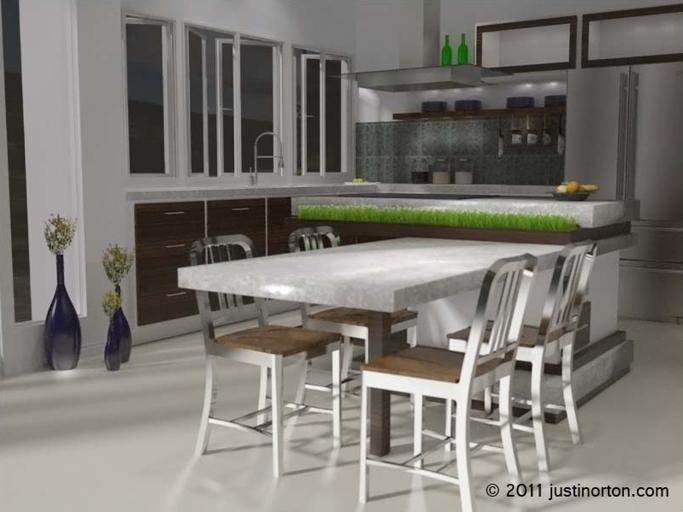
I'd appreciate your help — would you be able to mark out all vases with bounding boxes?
[103,317,120,371]
[105,286,131,363]
[44,255,81,371]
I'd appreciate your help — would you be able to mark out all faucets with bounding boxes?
[248,131,284,185]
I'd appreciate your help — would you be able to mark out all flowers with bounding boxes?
[100,289,122,316]
[39,212,79,254]
[100,239,136,286]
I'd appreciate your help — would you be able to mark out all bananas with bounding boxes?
[580,185,599,191]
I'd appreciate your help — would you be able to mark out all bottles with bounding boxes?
[440,33,470,65]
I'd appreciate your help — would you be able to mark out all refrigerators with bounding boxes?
[566,60,683,324]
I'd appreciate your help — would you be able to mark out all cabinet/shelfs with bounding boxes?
[267,196,291,258]
[134,200,205,325]
[205,197,266,311]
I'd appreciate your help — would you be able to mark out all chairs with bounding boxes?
[443,236,598,469]
[286,224,418,424]
[188,233,345,479]
[357,251,539,512]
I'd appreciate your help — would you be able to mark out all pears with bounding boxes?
[567,181,579,192]
